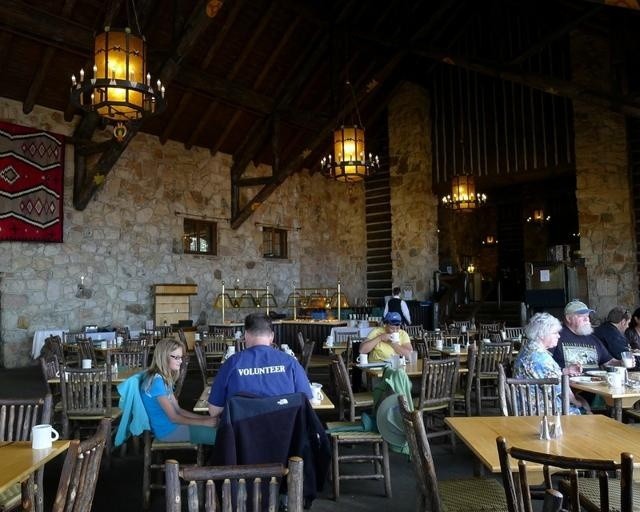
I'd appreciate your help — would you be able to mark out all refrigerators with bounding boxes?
[525,261,588,317]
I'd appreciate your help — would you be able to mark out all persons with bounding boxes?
[623,307,640,349]
[552,300,637,424]
[137,338,223,446]
[383,287,412,325]
[593,304,630,360]
[510,312,593,415]
[356,313,413,416]
[208,313,313,417]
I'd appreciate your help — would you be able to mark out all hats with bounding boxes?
[384,312,401,325]
[566,301,596,314]
[376,393,412,447]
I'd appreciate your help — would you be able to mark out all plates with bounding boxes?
[383,358,409,363]
[585,370,608,376]
[569,376,605,384]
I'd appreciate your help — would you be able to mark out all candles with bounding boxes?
[81,275,84,284]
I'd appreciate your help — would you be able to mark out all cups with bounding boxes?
[408,351,418,364]
[101,341,107,349]
[227,346,236,356]
[454,344,460,352]
[391,356,399,369]
[31,424,59,449]
[461,326,467,332]
[621,351,633,368]
[356,353,368,365]
[117,337,124,343]
[389,332,400,343]
[56,367,70,380]
[607,372,621,388]
[435,339,445,347]
[309,383,323,402]
[286,349,295,356]
[614,366,626,384]
[82,359,92,368]
[281,343,289,351]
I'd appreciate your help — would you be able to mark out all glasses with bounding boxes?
[623,309,630,319]
[170,355,183,360]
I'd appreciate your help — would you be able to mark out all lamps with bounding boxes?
[319,78,381,184]
[528,205,550,227]
[483,234,500,247]
[441,135,487,213]
[69,0,166,141]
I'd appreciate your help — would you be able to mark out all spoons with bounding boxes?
[626,370,633,387]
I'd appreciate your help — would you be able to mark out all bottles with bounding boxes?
[542,415,551,441]
[346,313,369,328]
[553,411,563,436]
